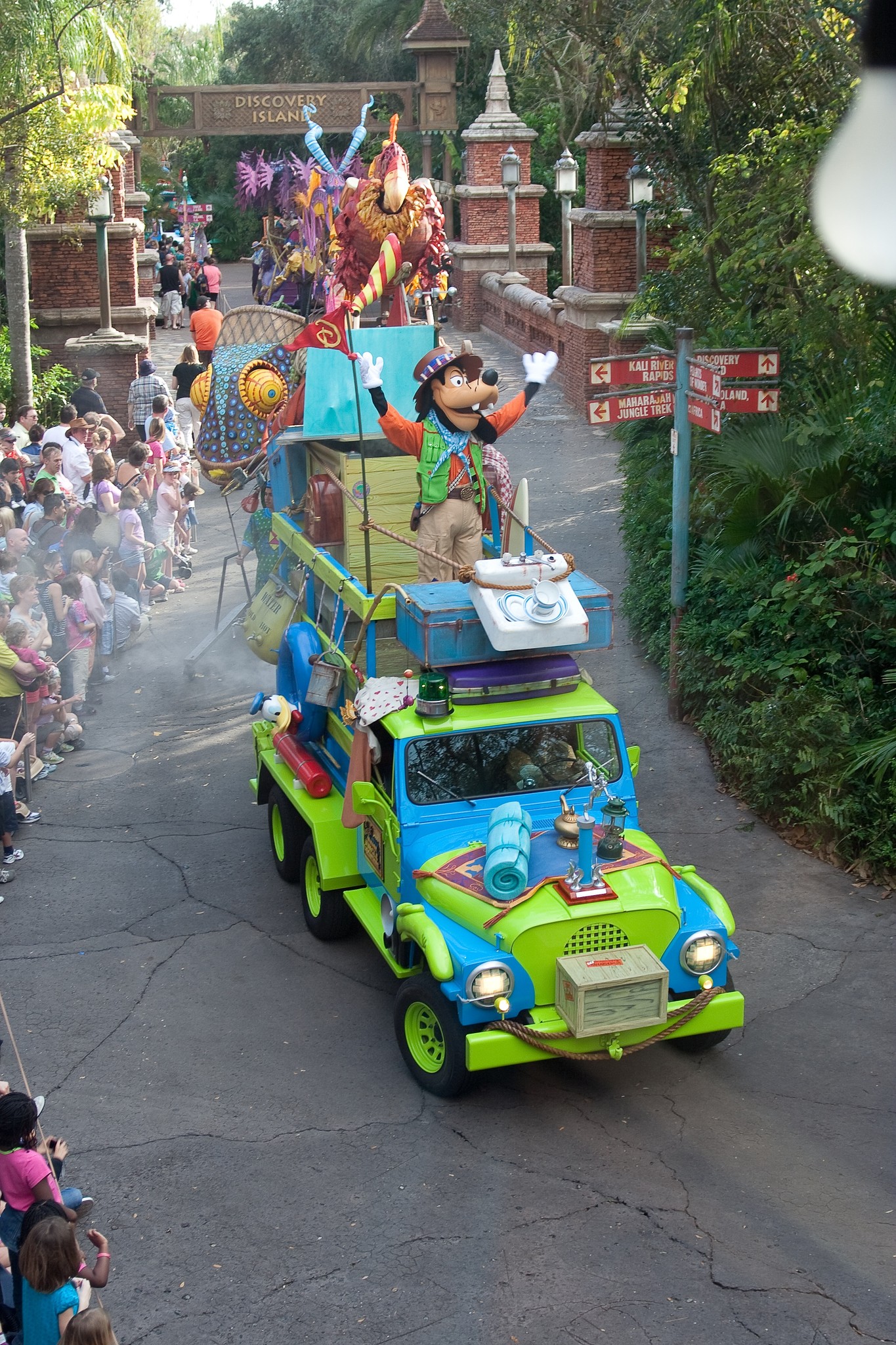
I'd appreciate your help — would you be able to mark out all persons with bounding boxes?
[239,211,303,295]
[0,234,223,904]
[0,1076,119,1345]
[236,478,278,600]
[500,727,585,792]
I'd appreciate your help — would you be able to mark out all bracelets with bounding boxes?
[96,1253,111,1259]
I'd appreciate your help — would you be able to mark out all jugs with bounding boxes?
[554,795,582,850]
[304,650,346,709]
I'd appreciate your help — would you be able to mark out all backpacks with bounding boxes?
[22,520,59,556]
[197,273,208,294]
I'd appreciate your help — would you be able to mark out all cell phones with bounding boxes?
[49,1140,61,1148]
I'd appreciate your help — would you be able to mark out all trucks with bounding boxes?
[249,503,748,1102]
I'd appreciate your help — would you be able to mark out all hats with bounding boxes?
[81,368,100,380]
[184,482,205,495]
[173,455,192,463]
[0,427,20,440]
[162,465,182,472]
[65,418,95,439]
[138,360,157,376]
[251,240,261,249]
[0,1092,45,1145]
[196,295,210,309]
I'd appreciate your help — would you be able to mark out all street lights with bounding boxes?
[625,154,658,322]
[550,145,580,309]
[494,142,529,279]
[90,174,118,336]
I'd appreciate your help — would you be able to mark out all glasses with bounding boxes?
[29,415,39,419]
[9,438,16,443]
[43,490,54,495]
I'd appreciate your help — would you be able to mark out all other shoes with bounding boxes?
[106,671,120,677]
[140,605,150,612]
[86,697,102,704]
[161,591,168,601]
[75,1197,94,1220]
[184,554,192,559]
[173,557,189,562]
[182,547,198,554]
[162,323,185,330]
[148,600,155,605]
[91,691,104,697]
[72,707,97,716]
[90,675,114,686]
[69,738,85,749]
[78,721,86,729]
[43,764,57,772]
[0,868,15,884]
[38,770,47,779]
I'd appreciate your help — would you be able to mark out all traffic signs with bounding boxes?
[694,348,780,377]
[588,360,676,386]
[176,204,213,213]
[177,214,213,224]
[689,365,723,400]
[587,390,674,424]
[685,397,722,435]
[695,389,781,413]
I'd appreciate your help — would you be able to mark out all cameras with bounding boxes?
[31,611,42,620]
[144,463,151,470]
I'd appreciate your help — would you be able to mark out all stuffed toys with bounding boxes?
[357,337,561,582]
[332,112,450,317]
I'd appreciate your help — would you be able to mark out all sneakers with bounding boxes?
[3,848,24,864]
[19,812,40,823]
[56,744,74,754]
[39,751,65,764]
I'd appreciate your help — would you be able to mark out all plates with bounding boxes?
[524,593,568,624]
[497,591,531,621]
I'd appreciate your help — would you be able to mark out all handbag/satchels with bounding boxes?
[92,509,121,548]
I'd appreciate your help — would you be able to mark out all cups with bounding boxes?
[530,578,561,614]
